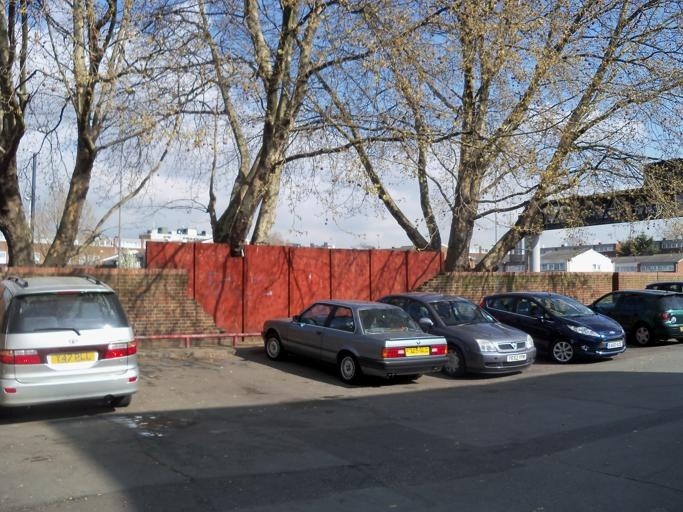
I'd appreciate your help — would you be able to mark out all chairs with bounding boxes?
[13,297,115,333]
[493,298,562,315]
[411,304,458,327]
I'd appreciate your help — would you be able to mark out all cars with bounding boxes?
[0,273,138,406]
